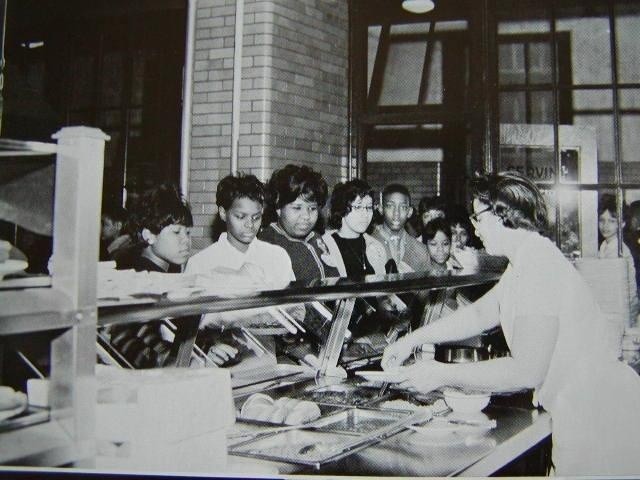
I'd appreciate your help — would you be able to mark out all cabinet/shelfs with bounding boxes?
[1,125,552,480]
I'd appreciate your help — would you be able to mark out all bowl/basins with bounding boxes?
[442,390,491,414]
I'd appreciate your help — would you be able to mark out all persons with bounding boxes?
[423,217,451,269]
[598,194,637,322]
[324,178,398,312]
[260,164,340,286]
[127,184,193,274]
[414,195,445,242]
[185,172,306,365]
[379,169,640,479]
[101,208,129,269]
[450,214,477,252]
[370,183,430,271]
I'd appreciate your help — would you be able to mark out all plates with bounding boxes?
[354,371,406,384]
[406,421,461,435]
[409,435,460,447]
[445,412,489,425]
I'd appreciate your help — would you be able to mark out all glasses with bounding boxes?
[354,204,372,212]
[469,206,490,227]
